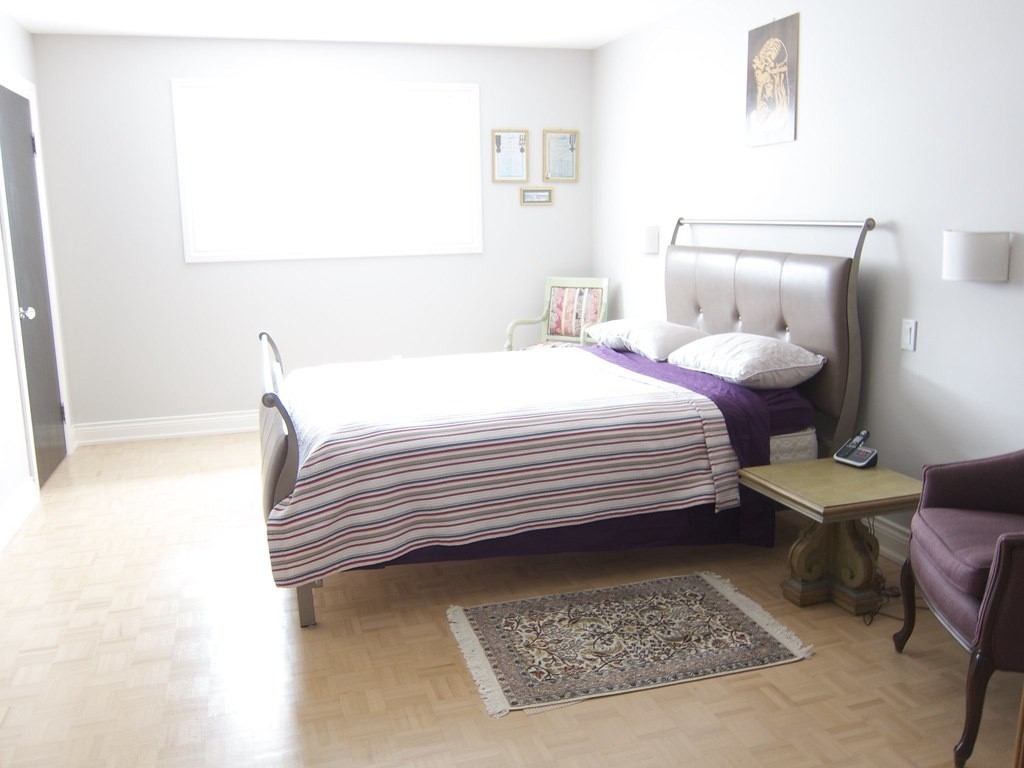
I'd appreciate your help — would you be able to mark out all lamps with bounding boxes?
[942,229,1010,282]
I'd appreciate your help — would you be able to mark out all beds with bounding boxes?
[258,217,876,627]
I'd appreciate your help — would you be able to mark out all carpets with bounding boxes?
[447,570,816,719]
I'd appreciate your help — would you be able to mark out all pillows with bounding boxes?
[668,332,827,389]
[586,319,710,362]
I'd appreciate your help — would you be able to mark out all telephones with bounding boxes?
[833,430,878,469]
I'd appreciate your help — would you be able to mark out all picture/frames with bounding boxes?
[492,129,529,183]
[520,187,553,205]
[543,128,579,183]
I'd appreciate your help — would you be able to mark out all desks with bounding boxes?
[738,458,924,614]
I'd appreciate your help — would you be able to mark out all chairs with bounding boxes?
[505,276,611,351]
[893,450,1024,768]
